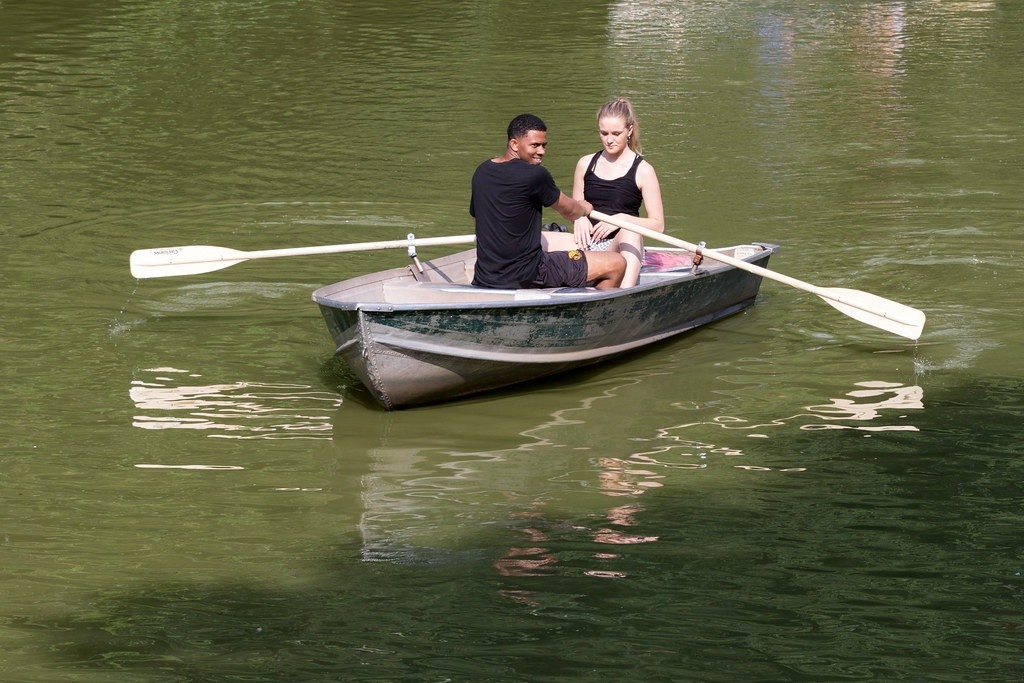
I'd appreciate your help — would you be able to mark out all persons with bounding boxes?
[575,98,664,288]
[469,114,627,292]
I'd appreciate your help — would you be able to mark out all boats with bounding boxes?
[312,229,781,412]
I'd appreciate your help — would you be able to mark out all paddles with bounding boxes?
[125,233,477,281]
[589,209,929,342]
